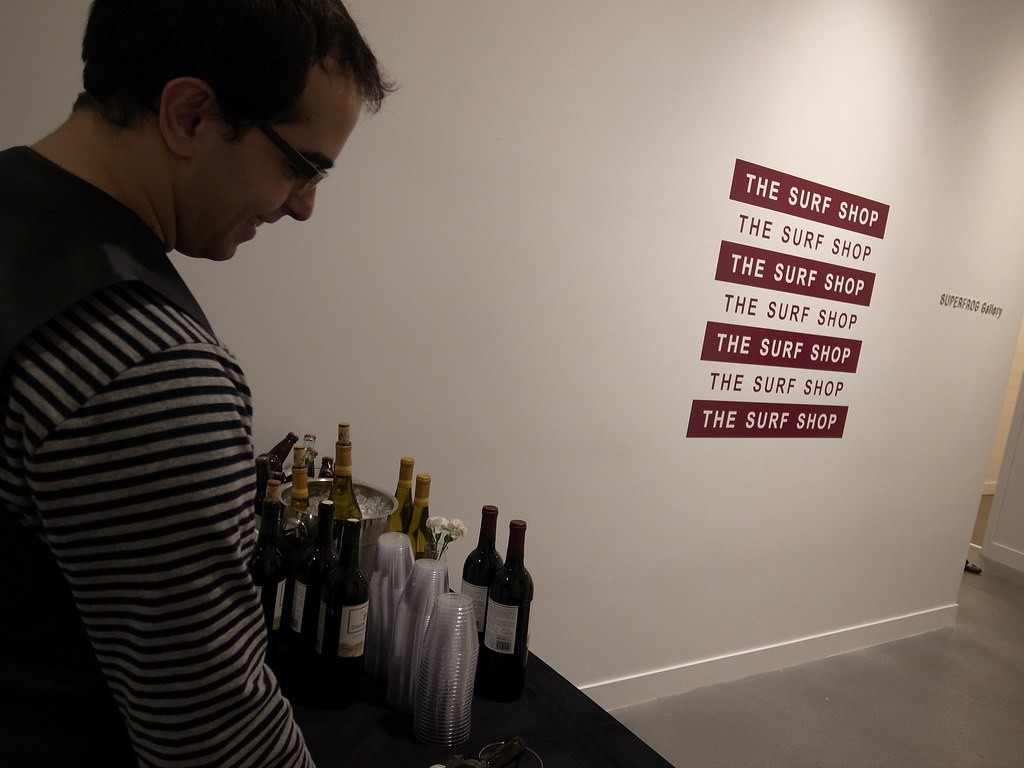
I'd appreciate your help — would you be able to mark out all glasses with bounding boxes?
[190,72,329,188]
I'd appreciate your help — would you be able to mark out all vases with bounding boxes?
[425,543,448,562]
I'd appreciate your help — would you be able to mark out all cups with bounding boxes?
[479,742,544,768]
[368,534,480,747]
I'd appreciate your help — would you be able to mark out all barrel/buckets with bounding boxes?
[278,478,399,579]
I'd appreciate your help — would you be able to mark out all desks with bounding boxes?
[267,645,674,768]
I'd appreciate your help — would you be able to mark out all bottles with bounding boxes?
[254,423,435,672]
[462,505,504,640]
[428,737,525,768]
[478,520,534,701]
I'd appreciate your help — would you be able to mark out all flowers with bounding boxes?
[425,515,467,562]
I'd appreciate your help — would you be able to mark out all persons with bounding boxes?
[0,0,386,768]
